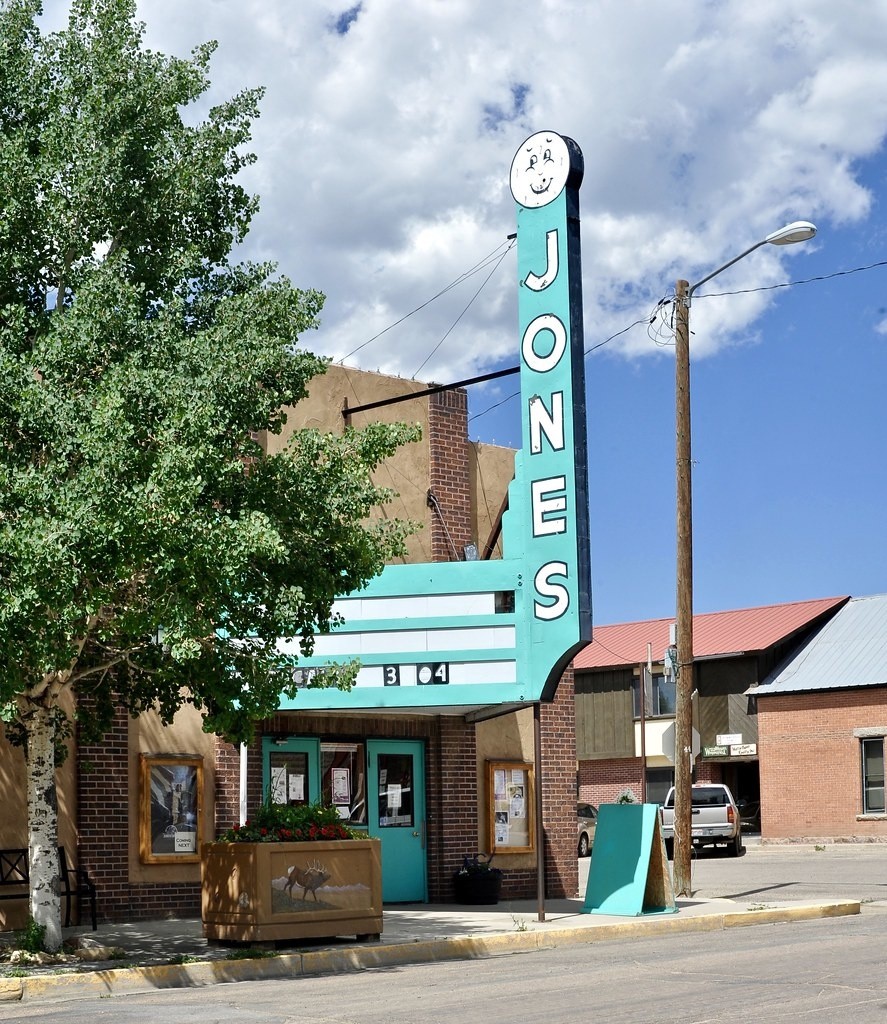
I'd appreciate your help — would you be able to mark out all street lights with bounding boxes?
[672,218,817,898]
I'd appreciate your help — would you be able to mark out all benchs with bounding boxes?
[0,846,98,932]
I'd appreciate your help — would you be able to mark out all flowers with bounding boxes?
[206,778,374,843]
[451,847,506,877]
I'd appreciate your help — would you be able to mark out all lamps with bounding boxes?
[463,541,482,561]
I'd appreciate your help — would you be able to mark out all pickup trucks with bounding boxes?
[660,778,748,860]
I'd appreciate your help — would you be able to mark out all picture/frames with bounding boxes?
[138,753,205,865]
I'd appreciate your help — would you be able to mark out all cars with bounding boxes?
[577,802,598,857]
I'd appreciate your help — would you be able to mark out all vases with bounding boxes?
[200,839,385,952]
[453,875,507,905]
